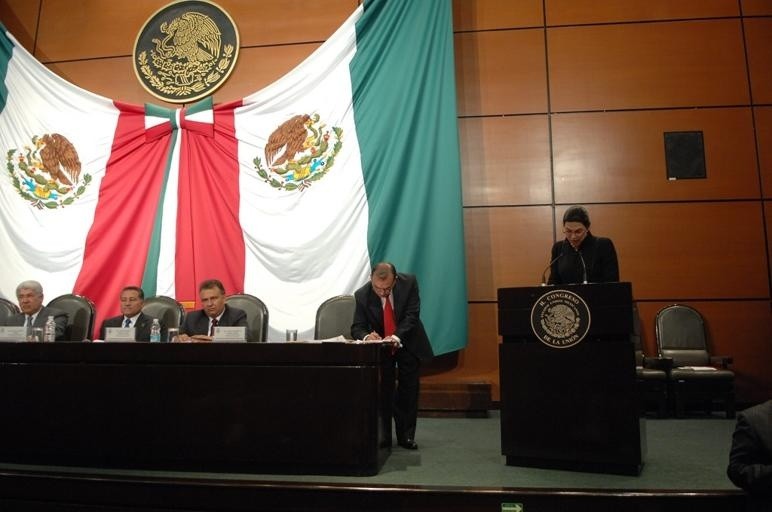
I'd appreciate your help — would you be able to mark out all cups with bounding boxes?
[169,327,180,342]
[32,328,44,341]
[286,329,297,343]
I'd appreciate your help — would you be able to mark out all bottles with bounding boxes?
[149,318,160,342]
[45,314,56,343]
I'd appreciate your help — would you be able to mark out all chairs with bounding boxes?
[143,295,185,338]
[631,302,674,416]
[47,293,98,341]
[225,292,270,342]
[655,305,738,416]
[0,299,21,325]
[315,295,360,339]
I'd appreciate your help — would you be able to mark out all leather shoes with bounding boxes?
[396,436,417,450]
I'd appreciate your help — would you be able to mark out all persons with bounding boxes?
[0,279,68,341]
[351,261,436,449]
[168,277,251,345]
[545,206,619,284]
[99,285,168,344]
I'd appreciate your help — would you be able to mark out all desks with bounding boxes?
[1,340,399,479]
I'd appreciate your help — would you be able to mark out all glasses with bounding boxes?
[562,229,588,236]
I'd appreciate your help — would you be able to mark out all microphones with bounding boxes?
[542,252,564,284]
[575,249,588,282]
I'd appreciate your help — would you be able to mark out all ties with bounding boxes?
[124,318,130,328]
[210,318,218,336]
[383,296,395,336]
[26,316,33,339]
